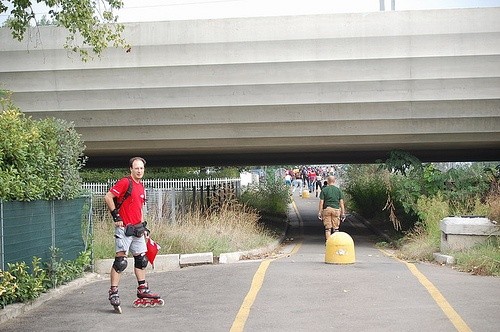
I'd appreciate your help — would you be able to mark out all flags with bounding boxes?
[145,238,161,264]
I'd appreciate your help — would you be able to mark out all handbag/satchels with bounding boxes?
[113,176,132,211]
[126,221,149,239]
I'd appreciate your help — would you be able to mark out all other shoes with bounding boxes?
[300,195,302,197]
[290,194,293,196]
[316,194,318,197]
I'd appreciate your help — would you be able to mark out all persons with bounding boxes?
[284,165,342,197]
[104,157,164,314]
[317,176,346,244]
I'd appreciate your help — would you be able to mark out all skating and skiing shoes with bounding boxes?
[108,286,124,314]
[132,284,165,308]
